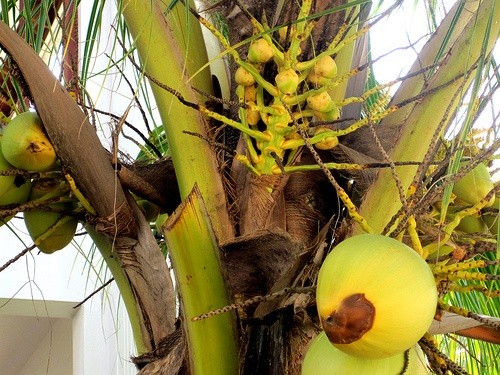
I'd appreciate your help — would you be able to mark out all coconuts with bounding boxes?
[231,36,499,375]
[0,109,80,254]
